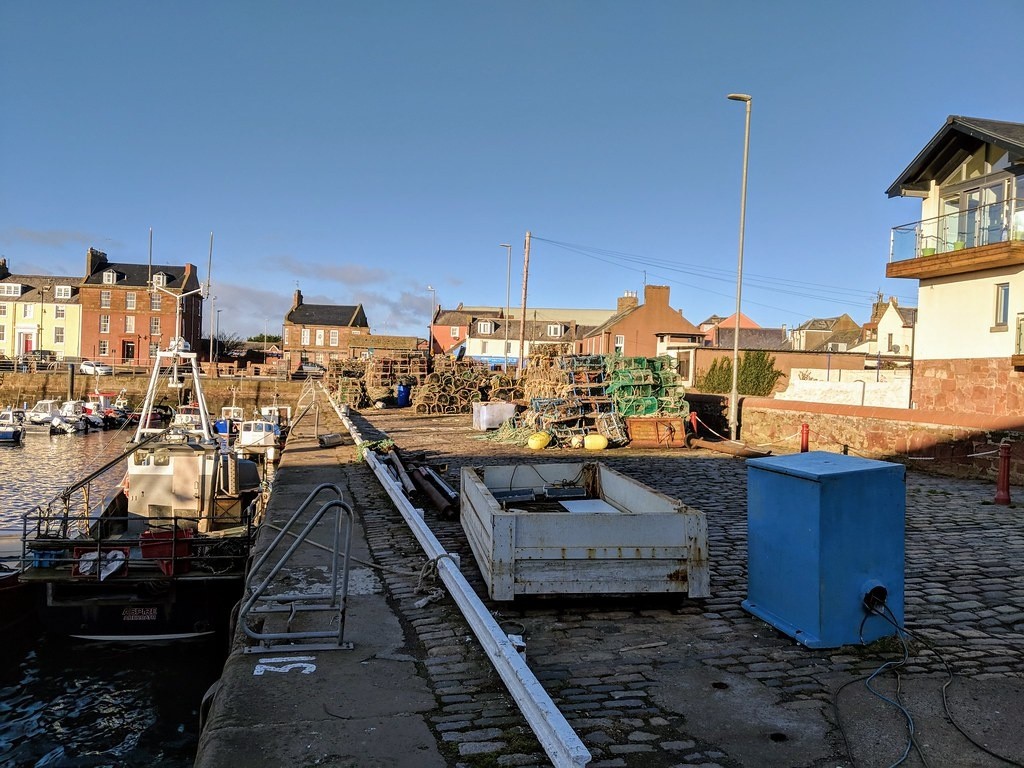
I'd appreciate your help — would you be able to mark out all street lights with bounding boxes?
[427,285,435,360]
[498,242,513,373]
[263,318,270,366]
[215,309,223,363]
[724,93,755,442]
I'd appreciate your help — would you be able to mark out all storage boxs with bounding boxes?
[31,548,65,568]
[140,529,195,558]
[74,546,130,579]
[471,400,517,432]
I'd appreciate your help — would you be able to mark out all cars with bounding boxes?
[302,360,326,377]
[79,360,112,376]
[0,355,13,369]
[17,349,56,364]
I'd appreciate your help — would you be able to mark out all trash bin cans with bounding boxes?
[398,384,410,406]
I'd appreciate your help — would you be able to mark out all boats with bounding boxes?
[0,342,293,462]
[16,280,264,642]
